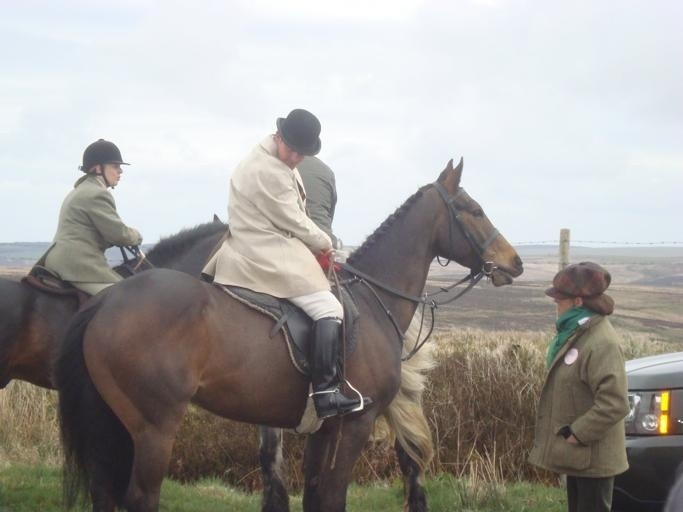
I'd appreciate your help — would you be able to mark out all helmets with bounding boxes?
[83,138,130,169]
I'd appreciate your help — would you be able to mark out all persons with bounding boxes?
[37,138,142,297]
[526,261,630,512]
[295,155,338,252]
[201,108,374,420]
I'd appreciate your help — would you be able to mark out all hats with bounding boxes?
[277,109,321,155]
[544,261,612,299]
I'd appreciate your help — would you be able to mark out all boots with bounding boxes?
[310,320,373,419]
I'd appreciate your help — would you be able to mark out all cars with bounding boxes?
[610,350,682,512]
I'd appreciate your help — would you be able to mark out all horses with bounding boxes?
[55,154,524,512]
[0,212,229,512]
